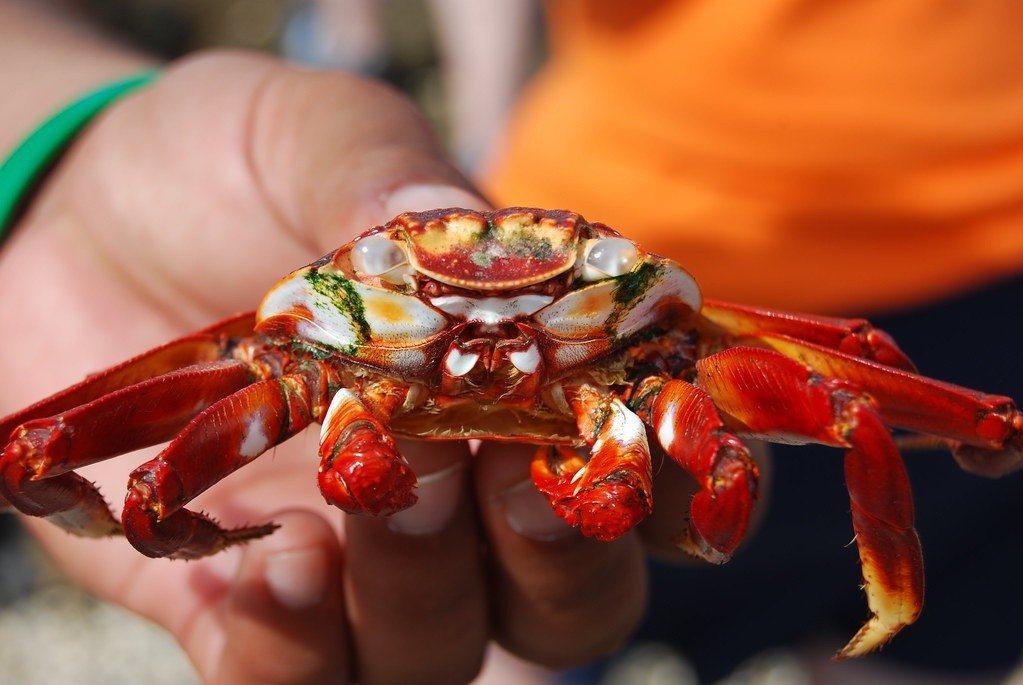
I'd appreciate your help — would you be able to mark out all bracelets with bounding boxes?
[0,67,165,244]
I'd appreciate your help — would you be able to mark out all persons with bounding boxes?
[0,0,772,685]
[282,1,1023,685]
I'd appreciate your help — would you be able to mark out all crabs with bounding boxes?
[0,207,1021,661]
